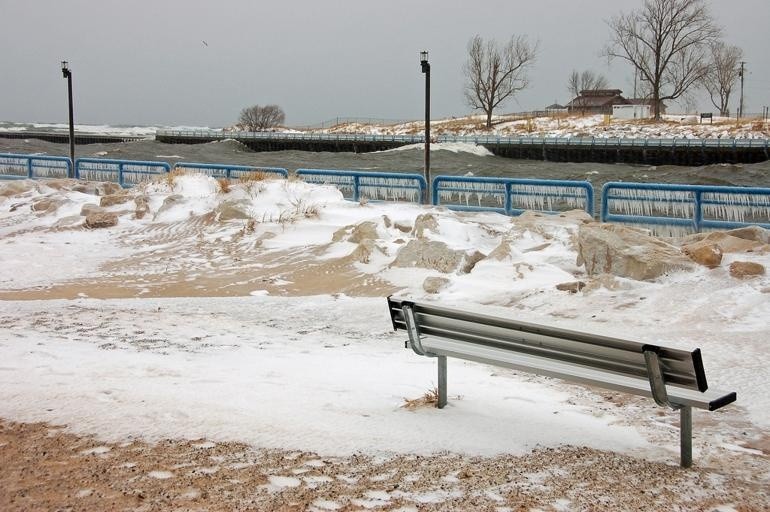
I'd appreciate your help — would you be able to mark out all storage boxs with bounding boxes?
[387,294,737,468]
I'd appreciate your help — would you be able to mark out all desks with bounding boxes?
[418,50,432,199]
[62,61,76,163]
